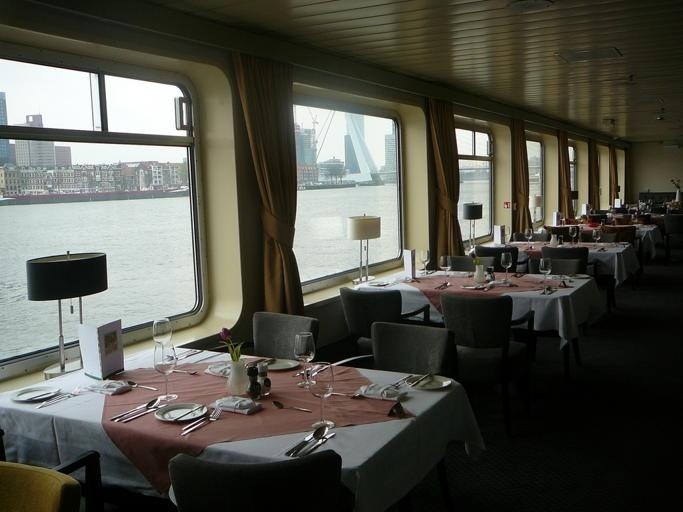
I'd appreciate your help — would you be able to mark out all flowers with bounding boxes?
[209,327,249,361]
[668,177,681,190]
[469,250,481,266]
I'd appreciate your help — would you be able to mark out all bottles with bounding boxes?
[257,365,271,395]
[247,366,261,399]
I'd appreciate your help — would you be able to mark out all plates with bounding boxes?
[459,284,487,290]
[11,384,62,402]
[367,282,389,286]
[153,401,209,423]
[255,358,301,372]
[405,374,453,390]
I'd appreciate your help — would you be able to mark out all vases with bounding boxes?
[474,266,486,287]
[226,356,251,395]
[675,188,681,210]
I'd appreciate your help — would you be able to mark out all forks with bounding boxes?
[34,384,81,409]
[178,405,222,438]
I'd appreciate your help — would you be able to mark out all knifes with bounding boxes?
[120,403,168,424]
[108,401,148,421]
[284,431,314,456]
[296,432,336,458]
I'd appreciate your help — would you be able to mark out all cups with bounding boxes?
[305,363,335,429]
[437,255,452,287]
[151,317,171,365]
[293,331,317,389]
[500,252,512,287]
[568,225,577,248]
[592,228,601,251]
[153,342,177,403]
[538,255,553,295]
[418,249,430,275]
[524,228,533,250]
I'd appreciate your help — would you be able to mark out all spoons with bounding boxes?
[271,399,312,413]
[125,381,160,392]
[171,369,197,375]
[114,398,162,423]
[181,408,216,431]
[288,425,332,459]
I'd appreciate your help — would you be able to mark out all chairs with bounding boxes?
[450,255,495,272]
[475,245,520,272]
[600,225,618,242]
[328,321,451,383]
[251,311,317,361]
[164,448,349,512]
[0,461,81,511]
[439,290,538,384]
[542,246,592,273]
[608,226,636,243]
[543,225,582,242]
[338,284,432,342]
[538,257,581,273]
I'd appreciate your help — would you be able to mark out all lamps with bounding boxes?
[24,253,108,380]
[527,194,543,234]
[462,202,483,251]
[344,215,381,286]
